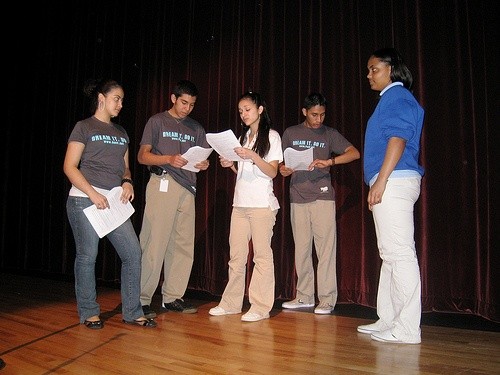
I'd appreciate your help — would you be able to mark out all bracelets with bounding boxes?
[330,157,335,166]
[121,179,135,187]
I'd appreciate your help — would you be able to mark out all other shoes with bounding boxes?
[124,318,156,328]
[84,318,102,329]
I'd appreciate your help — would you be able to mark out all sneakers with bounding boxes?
[208,306,241,315]
[315,306,334,314]
[282,299,314,308]
[164,300,197,313]
[357,323,377,334]
[242,313,270,321]
[141,304,156,318]
[370,330,406,344]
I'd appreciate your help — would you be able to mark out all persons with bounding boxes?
[63,79,159,329]
[356,47,425,345]
[278,94,361,314]
[207,91,283,322]
[136,80,210,319]
